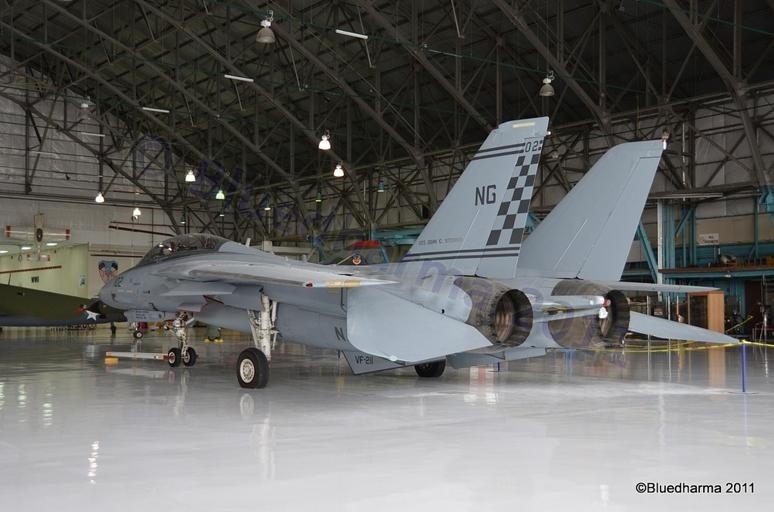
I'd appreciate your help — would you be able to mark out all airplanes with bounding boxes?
[0,283,127,335]
[92,114,746,390]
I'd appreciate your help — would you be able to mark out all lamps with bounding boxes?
[318,129,344,177]
[254,9,276,45]
[538,29,555,98]
[215,185,225,200]
